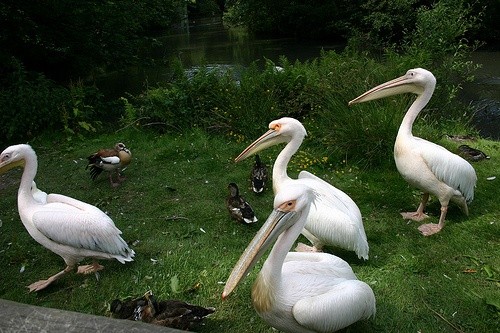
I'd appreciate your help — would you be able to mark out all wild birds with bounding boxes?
[348,67,478,237]
[453,144,487,163]
[236,116,369,261]
[250,154,270,195]
[107,289,217,331]
[222,182,377,332]
[1,143,136,294]
